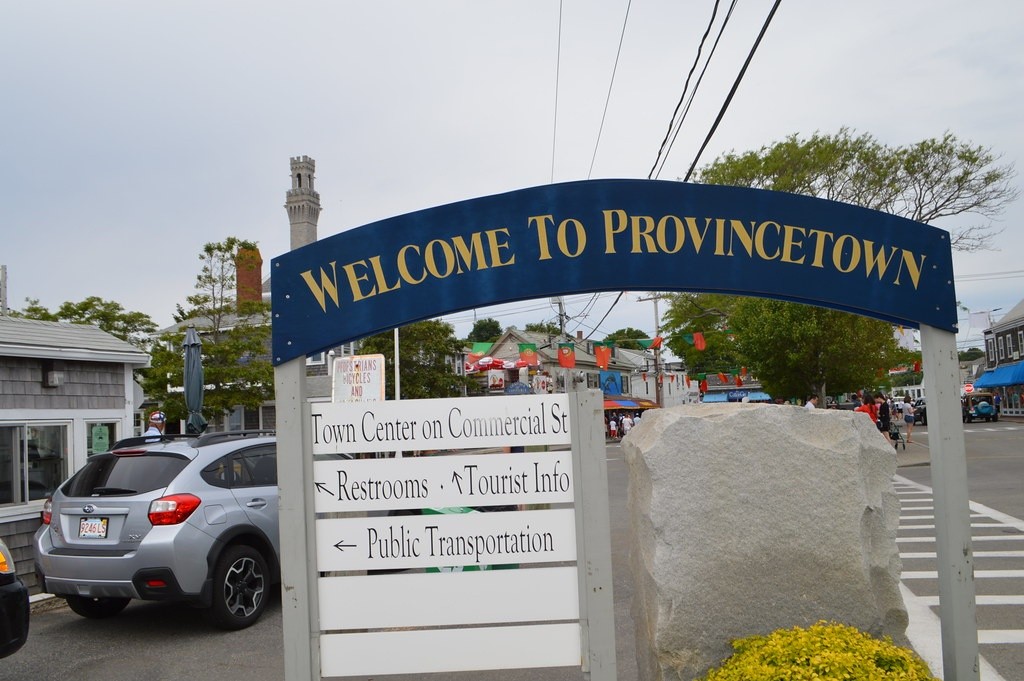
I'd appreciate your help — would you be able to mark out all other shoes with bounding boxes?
[908,439,914,443]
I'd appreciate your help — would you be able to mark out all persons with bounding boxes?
[994,392,1002,417]
[605,412,640,441]
[851,392,917,445]
[804,394,819,408]
[140,411,168,443]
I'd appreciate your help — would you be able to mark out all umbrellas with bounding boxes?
[181,324,209,438]
[465,356,541,387]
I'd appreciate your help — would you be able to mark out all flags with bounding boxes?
[467,332,706,371]
[696,367,747,392]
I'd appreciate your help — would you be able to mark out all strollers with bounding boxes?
[890,421,906,449]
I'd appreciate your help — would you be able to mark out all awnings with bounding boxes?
[702,394,728,402]
[748,392,772,400]
[604,399,661,410]
[973,360,1024,388]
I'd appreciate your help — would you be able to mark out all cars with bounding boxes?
[913,398,927,424]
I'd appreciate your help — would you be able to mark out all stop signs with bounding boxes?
[964,384,974,393]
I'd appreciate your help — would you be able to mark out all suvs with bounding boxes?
[893,397,905,411]
[34,429,354,629]
[962,393,999,422]
